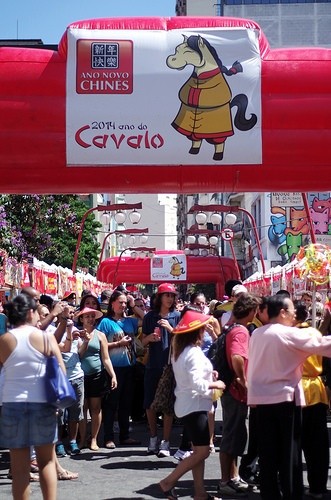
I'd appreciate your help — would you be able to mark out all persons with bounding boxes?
[0,281,331,500]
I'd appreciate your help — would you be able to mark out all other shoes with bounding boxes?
[58,469,78,480]
[7,470,40,482]
[105,444,116,449]
[30,465,39,472]
[172,449,191,464]
[120,440,140,445]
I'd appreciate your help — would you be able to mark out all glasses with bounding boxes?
[135,304,144,308]
[194,302,205,306]
[286,308,297,314]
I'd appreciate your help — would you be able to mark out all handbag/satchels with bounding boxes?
[45,356,77,409]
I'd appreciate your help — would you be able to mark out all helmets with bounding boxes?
[156,283,178,297]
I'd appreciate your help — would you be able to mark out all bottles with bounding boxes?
[154,325,162,342]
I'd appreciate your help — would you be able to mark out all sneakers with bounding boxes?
[68,442,80,454]
[56,443,68,457]
[147,435,160,455]
[231,476,258,492]
[158,439,170,457]
[217,478,251,499]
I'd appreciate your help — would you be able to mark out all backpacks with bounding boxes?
[207,323,242,393]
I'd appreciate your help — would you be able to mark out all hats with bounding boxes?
[231,284,247,297]
[61,290,76,301]
[73,306,103,323]
[171,311,214,335]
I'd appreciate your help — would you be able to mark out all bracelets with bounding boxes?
[66,337,72,341]
[118,341,120,347]
[62,316,68,320]
